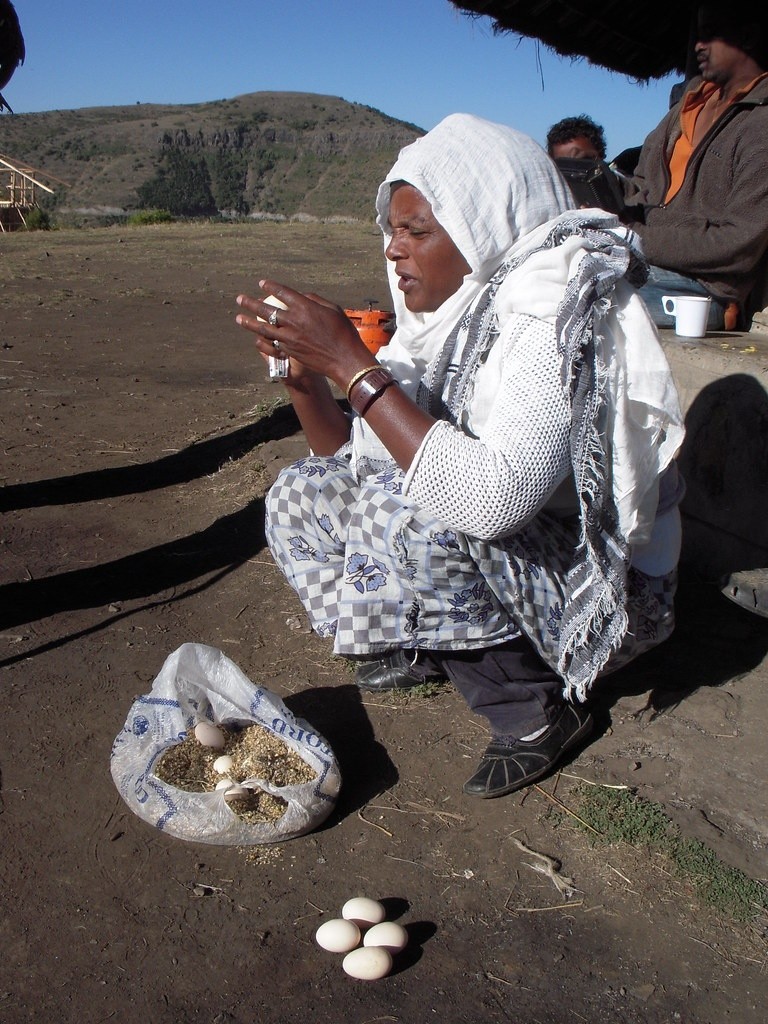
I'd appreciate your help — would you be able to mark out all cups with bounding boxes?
[662,296,712,338]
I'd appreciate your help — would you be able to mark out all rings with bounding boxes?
[272,339,280,352]
[268,308,281,327]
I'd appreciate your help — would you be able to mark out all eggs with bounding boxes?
[342,897,385,929]
[213,756,233,774]
[315,919,361,952]
[363,921,408,955]
[195,722,225,748]
[215,779,249,802]
[256,294,288,323]
[342,946,392,980]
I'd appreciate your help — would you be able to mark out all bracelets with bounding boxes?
[347,365,400,417]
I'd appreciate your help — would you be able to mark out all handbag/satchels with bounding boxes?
[110,644,342,844]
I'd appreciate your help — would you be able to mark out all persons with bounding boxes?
[548,0,768,332]
[235,112,686,797]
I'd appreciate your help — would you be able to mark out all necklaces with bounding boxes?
[712,99,720,122]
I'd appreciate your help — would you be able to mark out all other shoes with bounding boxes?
[464,700,593,799]
[354,651,432,690]
